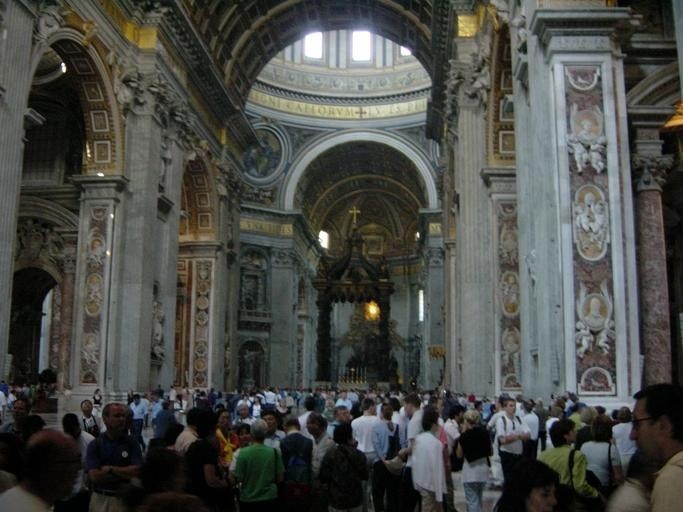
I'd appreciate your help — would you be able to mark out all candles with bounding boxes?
[338,365,368,377]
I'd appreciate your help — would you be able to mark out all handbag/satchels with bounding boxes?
[230,480,241,504]
[379,455,405,475]
[566,488,605,512]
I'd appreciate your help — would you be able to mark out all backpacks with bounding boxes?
[283,436,314,500]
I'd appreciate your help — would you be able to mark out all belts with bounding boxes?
[93,489,121,497]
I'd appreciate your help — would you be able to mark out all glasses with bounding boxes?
[630,413,653,428]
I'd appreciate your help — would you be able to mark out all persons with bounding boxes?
[496,200,519,380]
[628,383,682,511]
[1,378,636,512]
[566,134,616,360]
[79,237,106,368]
[149,299,166,362]
[252,131,271,177]
[241,348,262,388]
